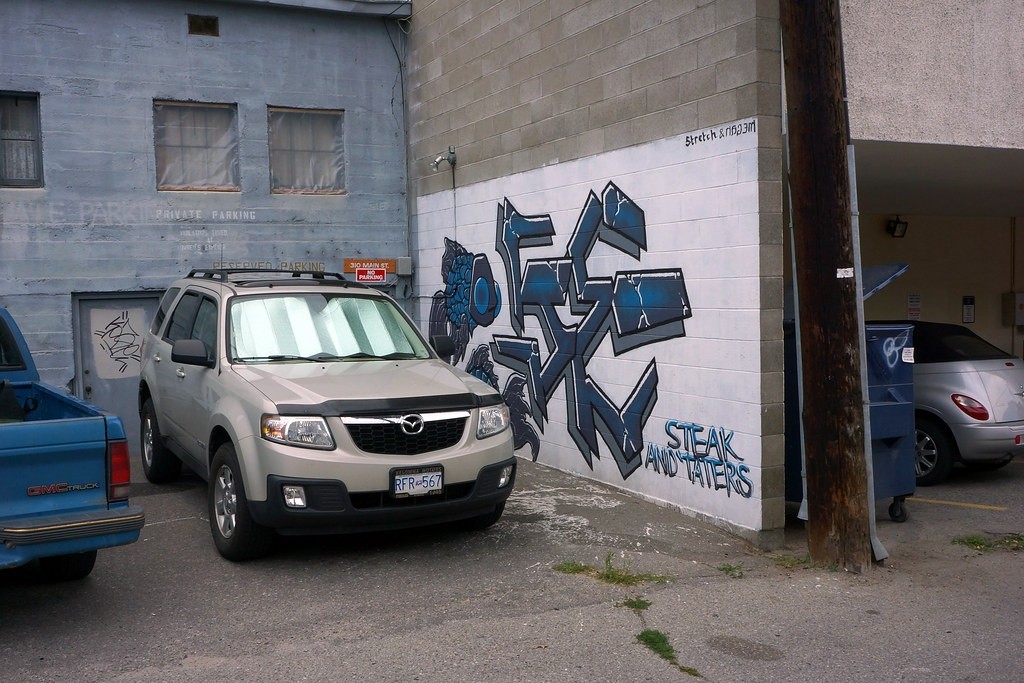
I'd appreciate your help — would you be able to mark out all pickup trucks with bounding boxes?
[0,308,146,578]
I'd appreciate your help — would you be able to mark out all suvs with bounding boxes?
[138,268,518,563]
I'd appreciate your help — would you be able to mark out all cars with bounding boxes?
[866,320,1024,487]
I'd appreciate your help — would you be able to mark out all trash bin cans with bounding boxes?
[783,264,916,523]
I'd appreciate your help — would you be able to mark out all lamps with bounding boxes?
[884,214,909,238]
[430,145,457,172]
